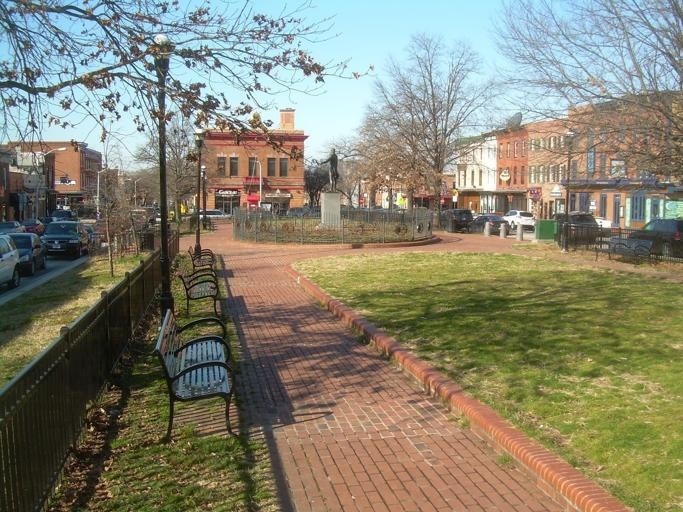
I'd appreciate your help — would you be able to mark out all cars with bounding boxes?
[627,219,683,258]
[550,211,599,241]
[199,209,232,220]
[131,203,161,217]
[439,209,537,235]
[0,210,94,289]
[286,207,320,217]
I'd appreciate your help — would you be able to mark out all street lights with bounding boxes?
[152,35,175,327]
[97,166,121,213]
[193,129,206,256]
[563,132,574,252]
[36,147,67,219]
[135,177,149,206]
[201,165,207,230]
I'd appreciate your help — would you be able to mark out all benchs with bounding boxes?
[599,235,657,266]
[151,307,236,438]
[179,244,223,315]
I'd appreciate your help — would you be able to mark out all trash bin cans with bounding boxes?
[535,219,559,240]
[139,233,155,251]
